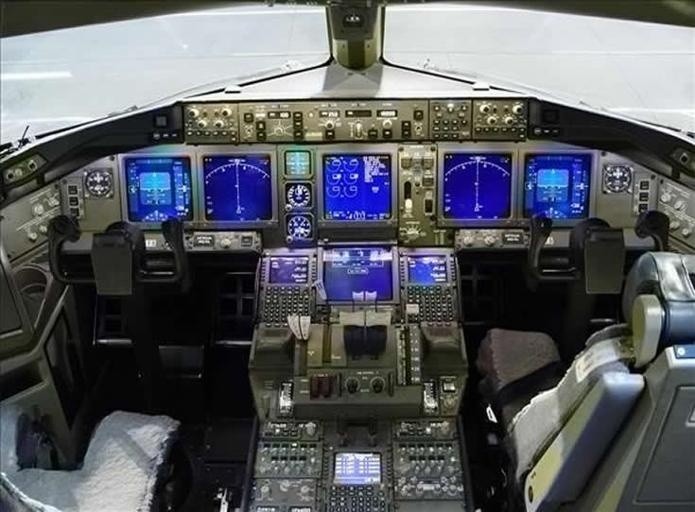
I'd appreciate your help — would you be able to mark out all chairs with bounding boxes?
[0,410,184,509]
[481,256,693,509]
